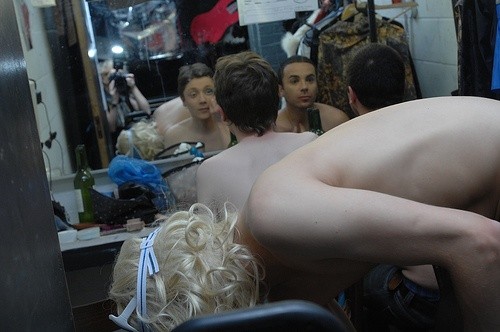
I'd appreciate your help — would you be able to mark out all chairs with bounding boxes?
[175,299,346,332]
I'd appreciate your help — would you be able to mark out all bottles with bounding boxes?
[73,146,94,222]
[312,109,325,136]
[229,130,237,148]
[306,108,313,132]
[78,144,94,190]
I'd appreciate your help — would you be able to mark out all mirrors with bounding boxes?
[70,0,258,172]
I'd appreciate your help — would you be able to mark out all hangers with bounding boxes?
[302,0,383,25]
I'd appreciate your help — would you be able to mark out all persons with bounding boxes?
[195,52,324,215]
[108,96,500,332]
[345,43,442,329]
[99,60,150,131]
[115,84,287,161]
[272,56,349,133]
[163,63,232,155]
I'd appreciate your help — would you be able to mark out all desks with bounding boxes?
[57,227,140,332]
